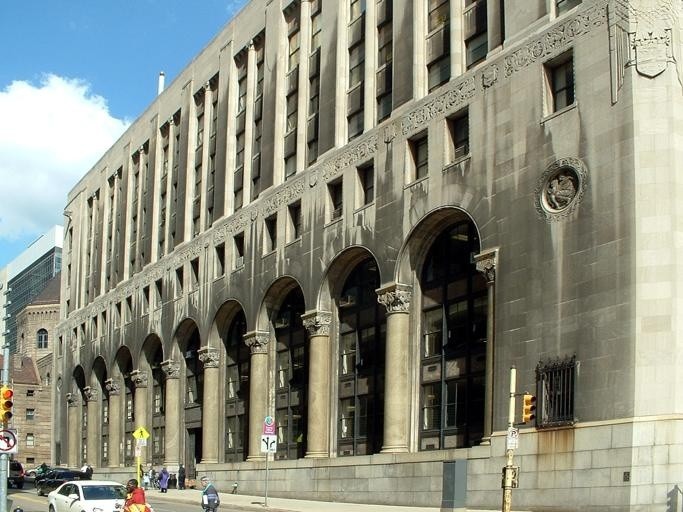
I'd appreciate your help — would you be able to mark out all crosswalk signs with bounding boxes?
[132,427,149,446]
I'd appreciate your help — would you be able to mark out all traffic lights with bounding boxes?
[0,387,14,421]
[522,394,536,422]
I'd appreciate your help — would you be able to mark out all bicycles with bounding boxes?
[153,476,160,490]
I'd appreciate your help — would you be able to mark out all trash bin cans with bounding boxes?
[167,479,175,489]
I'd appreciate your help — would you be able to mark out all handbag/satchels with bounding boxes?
[200,494,220,509]
[158,473,163,480]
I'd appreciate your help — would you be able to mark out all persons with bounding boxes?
[140,463,186,493]
[80,462,88,472]
[200,476,219,512]
[119,479,151,512]
[85,465,93,480]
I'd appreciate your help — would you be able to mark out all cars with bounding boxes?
[7,460,154,512]
